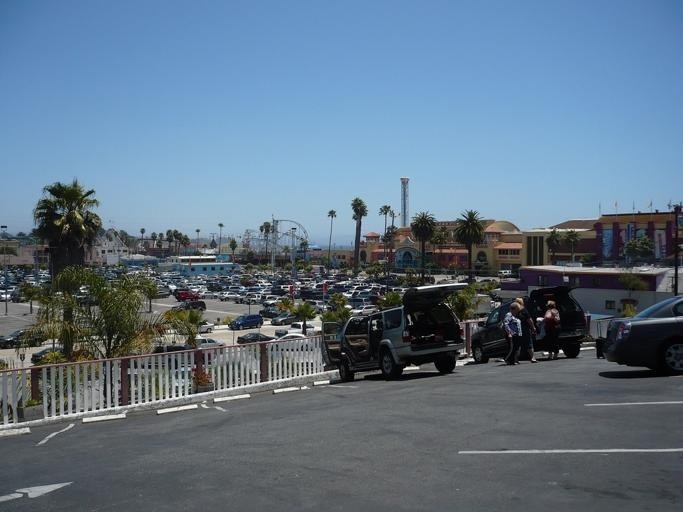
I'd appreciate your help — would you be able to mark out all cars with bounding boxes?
[418,269,518,284]
[594,295,682,378]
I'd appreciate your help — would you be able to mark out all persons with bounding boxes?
[501,300,523,366]
[539,300,560,361]
[509,298,537,363]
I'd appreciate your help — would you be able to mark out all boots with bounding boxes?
[528,348,537,362]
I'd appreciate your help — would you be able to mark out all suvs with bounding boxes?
[319,281,470,380]
[469,284,587,365]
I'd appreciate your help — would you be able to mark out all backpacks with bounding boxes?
[549,309,561,325]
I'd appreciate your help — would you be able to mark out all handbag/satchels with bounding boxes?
[495,320,506,337]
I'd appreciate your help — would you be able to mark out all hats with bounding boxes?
[513,298,523,304]
[545,300,555,307]
